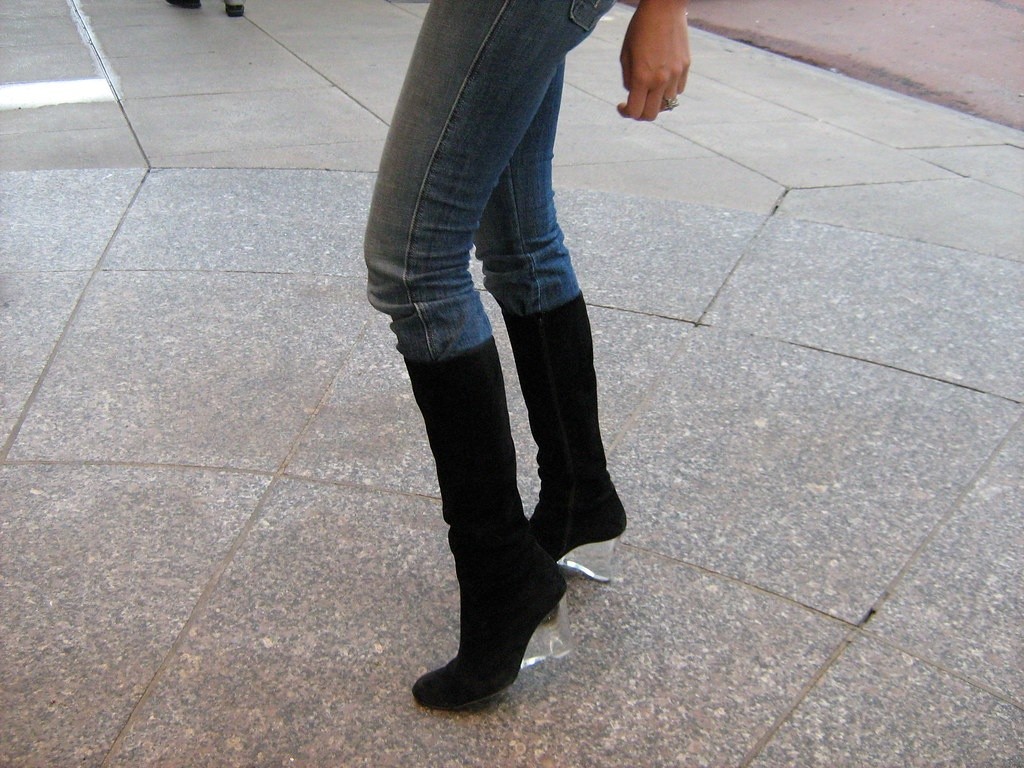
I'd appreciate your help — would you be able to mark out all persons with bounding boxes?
[166,0,247,17]
[364,0,691,715]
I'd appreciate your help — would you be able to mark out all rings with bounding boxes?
[663,96,680,110]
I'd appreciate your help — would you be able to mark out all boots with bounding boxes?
[501,292,628,582]
[404,335,568,714]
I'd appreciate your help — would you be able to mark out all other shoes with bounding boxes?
[226,5,244,17]
[166,0,201,9]
